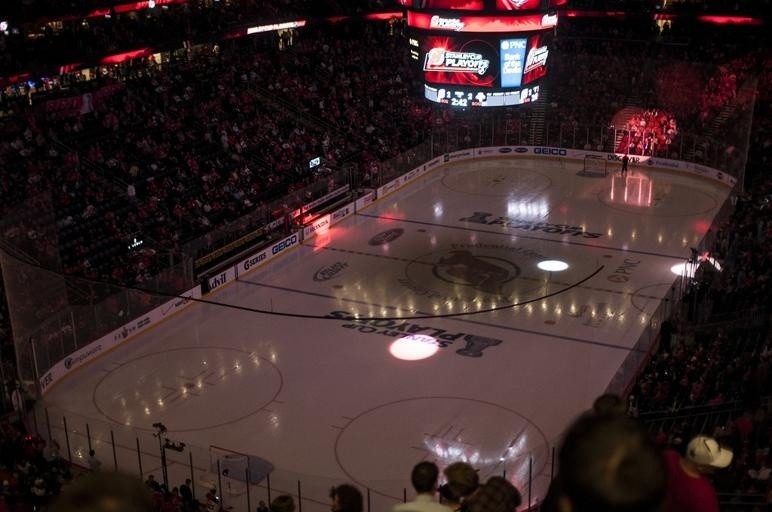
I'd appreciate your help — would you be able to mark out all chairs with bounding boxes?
[0,42,424,322]
[638,156,769,419]
[425,44,762,156]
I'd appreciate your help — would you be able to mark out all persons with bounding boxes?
[0,244,101,459]
[548,408,672,510]
[1,457,524,511]
[398,0,770,181]
[102,243,227,339]
[592,394,625,420]
[0,0,397,244]
[622,181,770,511]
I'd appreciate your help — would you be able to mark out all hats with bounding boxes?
[445,462,478,485]
[687,437,733,467]
